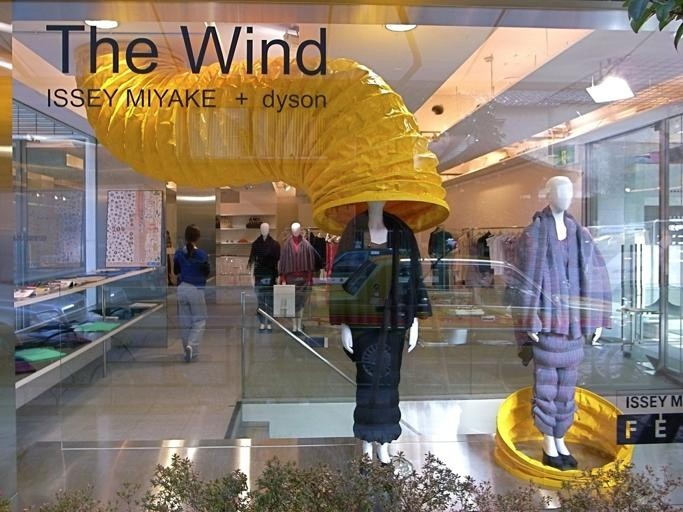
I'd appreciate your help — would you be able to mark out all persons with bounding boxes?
[174,224,211,362]
[279,222,316,333]
[329,199,432,466]
[247,222,281,333]
[504,174,613,468]
[428,225,456,290]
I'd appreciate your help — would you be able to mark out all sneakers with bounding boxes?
[543,447,577,470]
[183,346,200,362]
[360,451,392,475]
[259,328,272,332]
[292,330,302,335]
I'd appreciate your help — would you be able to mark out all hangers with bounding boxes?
[458,229,523,243]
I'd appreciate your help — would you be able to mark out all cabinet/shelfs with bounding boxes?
[216,213,276,275]
[14,267,163,409]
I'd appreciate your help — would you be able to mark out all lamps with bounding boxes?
[284,26,300,45]
